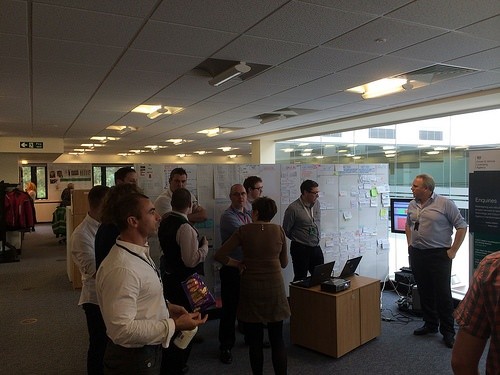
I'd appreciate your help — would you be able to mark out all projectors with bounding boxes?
[320,278,352,292]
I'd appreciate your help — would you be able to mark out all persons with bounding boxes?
[218,184,272,364]
[151,168,208,343]
[214,196,289,375]
[450,248,500,375]
[113,167,138,188]
[158,188,210,374]
[96,188,210,375]
[94,181,175,375]
[282,180,325,284]
[61,183,74,210]
[405,174,467,348]
[25,182,37,233]
[69,184,109,348]
[242,175,271,330]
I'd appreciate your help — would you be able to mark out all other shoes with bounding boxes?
[443,335,455,348]
[413,325,438,335]
[182,365,189,371]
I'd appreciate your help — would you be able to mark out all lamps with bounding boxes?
[173,140,186,145]
[208,62,251,87]
[147,106,168,120]
[362,79,413,101]
[207,129,221,137]
[259,115,286,124]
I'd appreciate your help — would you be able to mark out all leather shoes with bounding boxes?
[219,348,232,364]
[245,336,270,349]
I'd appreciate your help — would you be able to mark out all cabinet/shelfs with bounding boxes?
[288,273,382,359]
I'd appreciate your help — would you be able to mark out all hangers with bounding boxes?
[13,187,19,192]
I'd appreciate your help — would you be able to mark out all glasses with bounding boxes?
[254,186,263,190]
[310,191,318,194]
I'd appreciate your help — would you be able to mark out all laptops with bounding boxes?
[332,256,363,279]
[290,261,335,288]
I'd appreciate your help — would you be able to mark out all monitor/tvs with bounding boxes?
[390,197,412,233]
